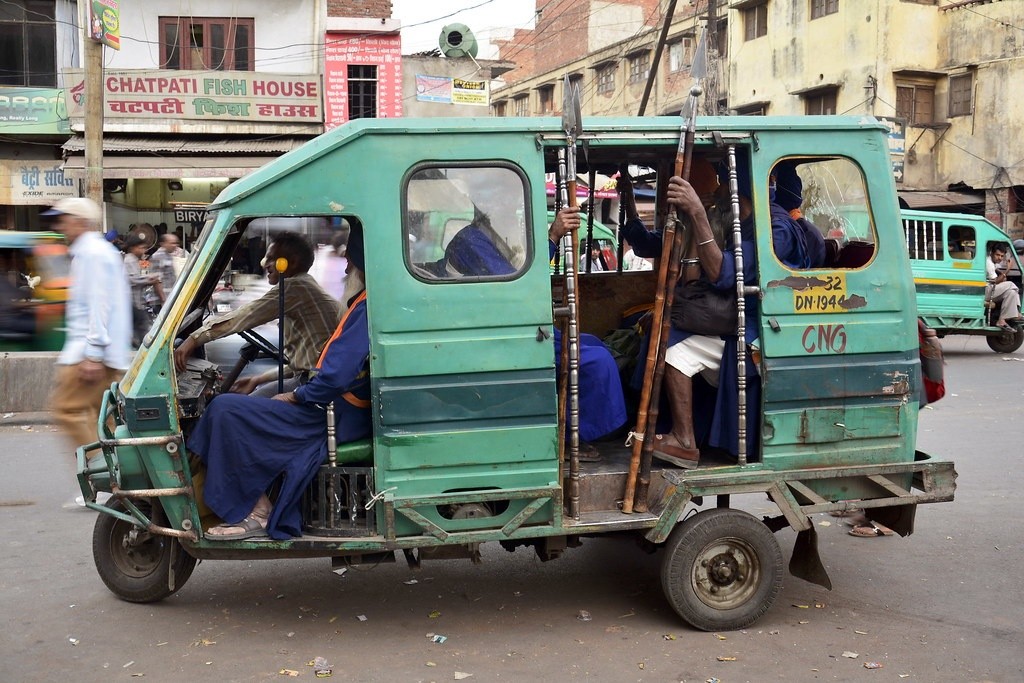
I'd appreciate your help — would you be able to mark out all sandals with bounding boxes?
[564,440,602,461]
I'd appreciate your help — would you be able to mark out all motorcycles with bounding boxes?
[423,209,628,272]
[805,205,1024,353]
[76,114,959,633]
[0,230,66,337]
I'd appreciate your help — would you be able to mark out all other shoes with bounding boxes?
[73,491,113,509]
[628,427,700,469]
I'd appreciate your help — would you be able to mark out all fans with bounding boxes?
[127,220,158,254]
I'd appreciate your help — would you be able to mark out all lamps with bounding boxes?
[167,181,183,190]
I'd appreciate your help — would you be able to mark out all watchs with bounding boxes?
[293,387,300,401]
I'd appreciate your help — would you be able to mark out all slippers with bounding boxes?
[999,323,1018,333]
[203,515,268,541]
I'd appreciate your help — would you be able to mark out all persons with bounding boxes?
[173,232,344,398]
[37,196,134,508]
[986,242,1024,334]
[580,240,603,272]
[106,226,191,343]
[185,221,373,544]
[443,168,628,460]
[615,155,845,470]
[623,237,652,270]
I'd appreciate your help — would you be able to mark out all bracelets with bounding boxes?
[681,256,699,263]
[697,238,715,247]
[1001,272,1006,278]
[681,261,700,265]
[548,236,557,247]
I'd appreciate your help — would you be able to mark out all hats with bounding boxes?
[125,233,147,246]
[710,150,776,196]
[345,226,365,269]
[36,198,103,221]
[105,229,118,241]
